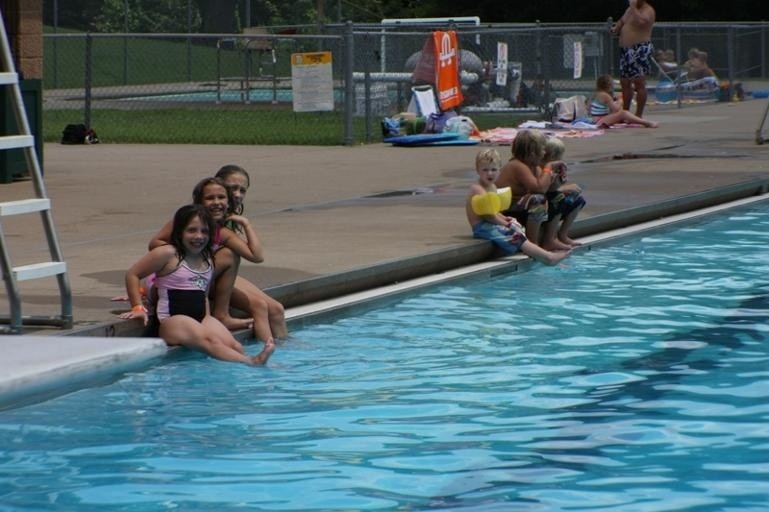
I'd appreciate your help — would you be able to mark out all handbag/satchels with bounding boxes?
[62,124,98,144]
[382,111,475,135]
[555,96,586,122]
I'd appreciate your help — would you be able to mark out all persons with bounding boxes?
[465,129,585,266]
[106,166,288,368]
[609,0,657,123]
[589,75,660,129]
[655,48,720,83]
